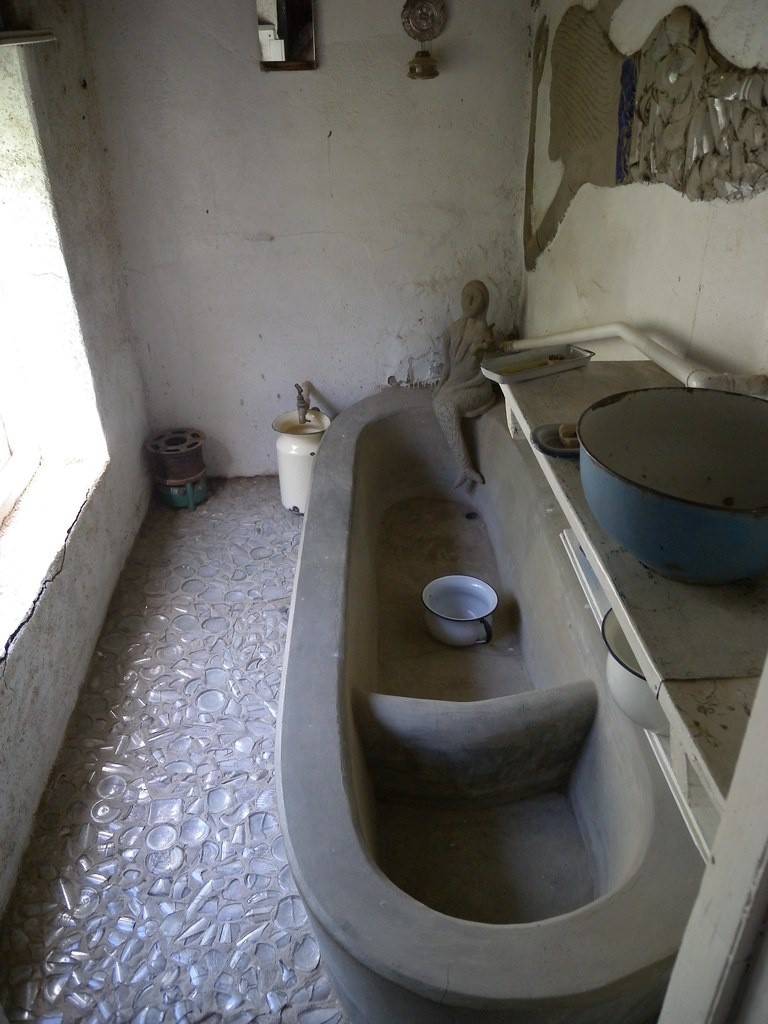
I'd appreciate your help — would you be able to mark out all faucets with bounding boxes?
[293,381,312,427]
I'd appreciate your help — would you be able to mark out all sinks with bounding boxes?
[270,407,332,517]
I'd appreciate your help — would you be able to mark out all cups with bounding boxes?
[421,574,499,649]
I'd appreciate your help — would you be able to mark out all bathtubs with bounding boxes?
[271,379,710,1024]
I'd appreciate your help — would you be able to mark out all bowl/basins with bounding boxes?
[575,386,768,586]
[601,607,670,738]
